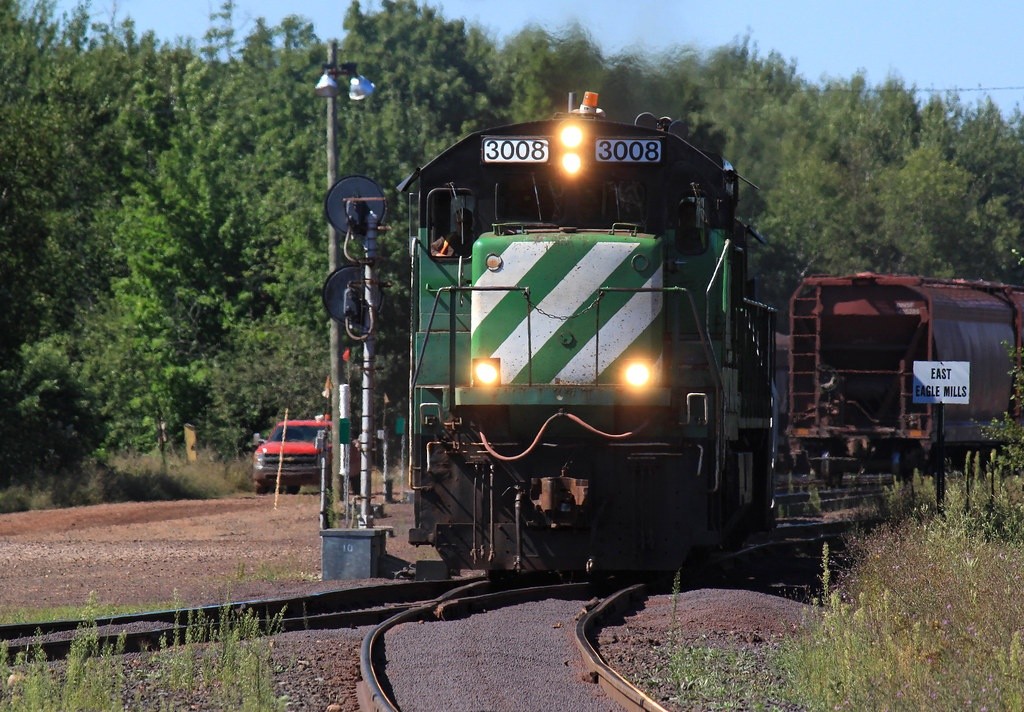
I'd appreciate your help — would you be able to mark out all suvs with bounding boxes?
[252,418,359,496]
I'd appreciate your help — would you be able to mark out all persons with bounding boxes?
[431,209,472,257]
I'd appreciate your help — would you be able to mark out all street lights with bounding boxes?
[312,37,377,530]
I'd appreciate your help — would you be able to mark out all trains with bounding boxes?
[394,90,1023,585]
